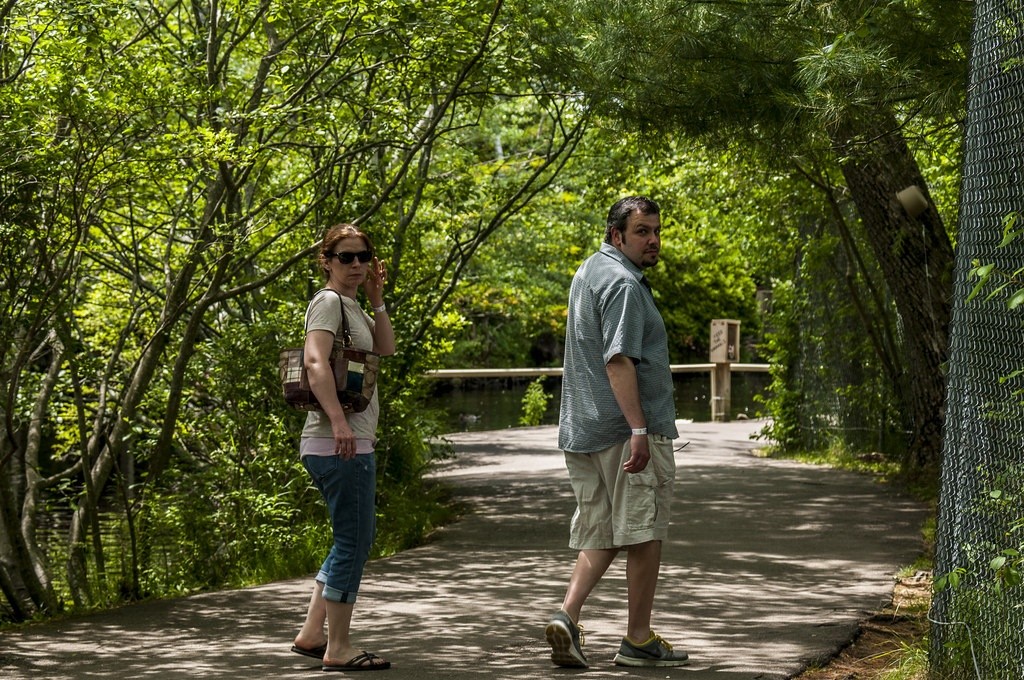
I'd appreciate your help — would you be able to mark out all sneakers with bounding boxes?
[613,630,689,667]
[545,609,589,668]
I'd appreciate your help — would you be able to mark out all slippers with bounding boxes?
[291,639,327,659]
[322,651,390,671]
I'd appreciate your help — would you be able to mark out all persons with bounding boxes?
[291,223,396,671]
[546,196,688,668]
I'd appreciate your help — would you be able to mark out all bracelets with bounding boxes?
[632,428,647,435]
[371,303,386,313]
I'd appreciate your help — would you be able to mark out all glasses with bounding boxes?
[323,251,372,264]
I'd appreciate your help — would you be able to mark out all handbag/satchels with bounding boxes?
[279,288,380,413]
